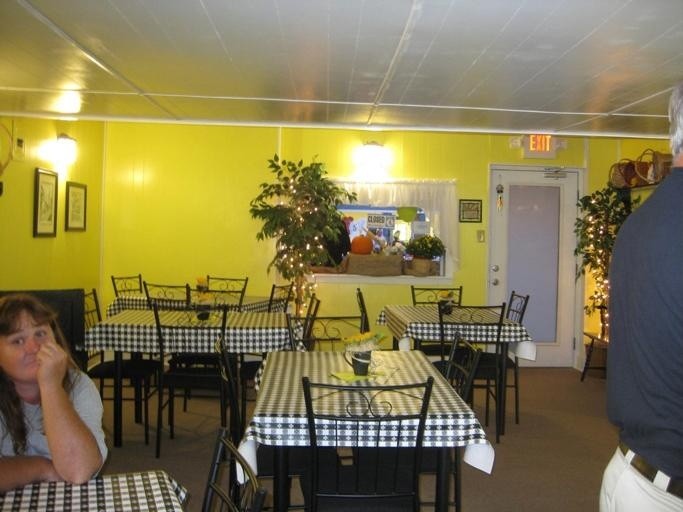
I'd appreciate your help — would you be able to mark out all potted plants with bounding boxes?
[402,233,444,276]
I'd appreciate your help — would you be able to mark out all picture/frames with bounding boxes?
[62,179,89,234]
[458,198,484,225]
[32,164,59,237]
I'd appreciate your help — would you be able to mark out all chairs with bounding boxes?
[0,273,539,512]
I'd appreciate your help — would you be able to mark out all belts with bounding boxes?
[615,438,683,501]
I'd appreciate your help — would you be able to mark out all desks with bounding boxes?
[578,330,608,384]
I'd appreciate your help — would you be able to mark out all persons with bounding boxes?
[598,79,683,512]
[0,293,109,494]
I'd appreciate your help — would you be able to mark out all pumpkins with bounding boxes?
[350,234,372,254]
